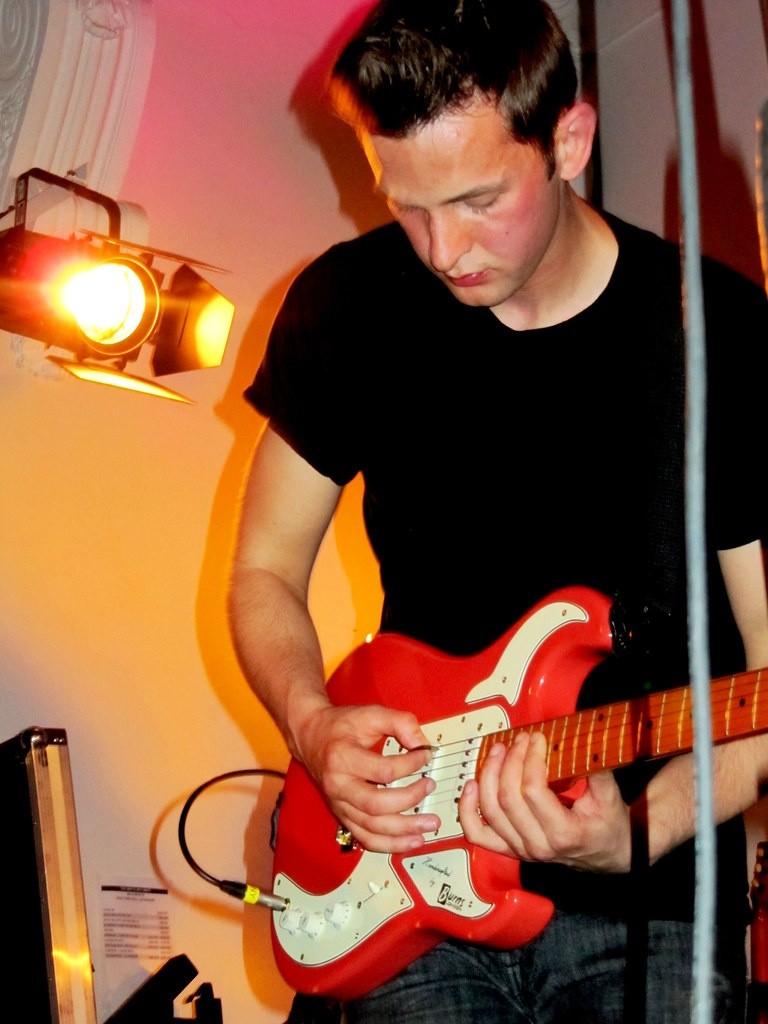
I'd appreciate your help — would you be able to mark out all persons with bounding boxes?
[227,0,768,1024]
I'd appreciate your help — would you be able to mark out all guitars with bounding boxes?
[270,584,768,1001]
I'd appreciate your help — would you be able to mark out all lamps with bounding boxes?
[1,166,235,408]
